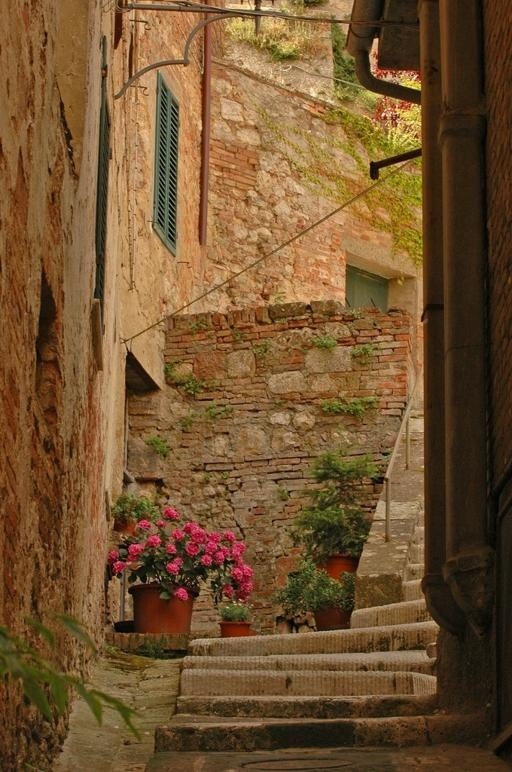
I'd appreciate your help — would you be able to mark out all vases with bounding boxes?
[128,582,197,635]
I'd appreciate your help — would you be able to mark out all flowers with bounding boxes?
[107,504,253,602]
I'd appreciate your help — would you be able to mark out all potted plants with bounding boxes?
[216,603,253,638]
[279,445,381,631]
